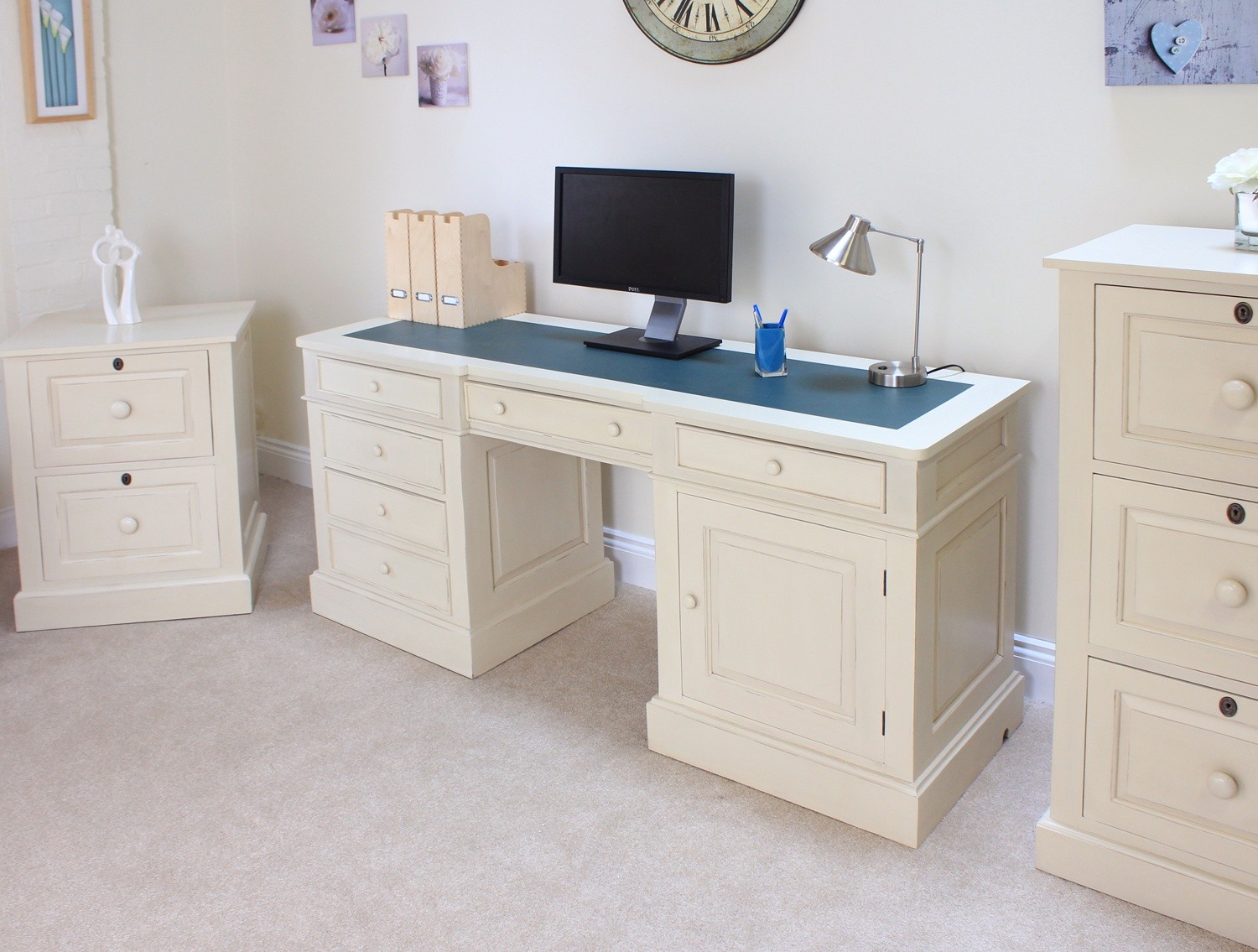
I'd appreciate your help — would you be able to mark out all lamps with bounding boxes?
[811,214,931,389]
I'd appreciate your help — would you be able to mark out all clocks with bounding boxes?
[622,1,803,65]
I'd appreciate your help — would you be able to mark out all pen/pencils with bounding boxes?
[778,308,788,329]
[753,310,763,329]
[753,304,763,321]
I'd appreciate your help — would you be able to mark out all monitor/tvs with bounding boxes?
[552,168,736,359]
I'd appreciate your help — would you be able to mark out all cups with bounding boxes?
[1234,192,1258,253]
[751,324,788,379]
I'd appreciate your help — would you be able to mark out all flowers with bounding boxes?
[418,48,462,80]
[1208,147,1258,188]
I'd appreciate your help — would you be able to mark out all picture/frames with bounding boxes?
[19,0,95,123]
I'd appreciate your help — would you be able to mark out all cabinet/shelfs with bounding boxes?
[294,311,1032,851]
[1033,225,1256,952]
[1,299,268,633]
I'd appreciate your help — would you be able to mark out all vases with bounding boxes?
[1234,193,1258,251]
[431,78,448,105]
